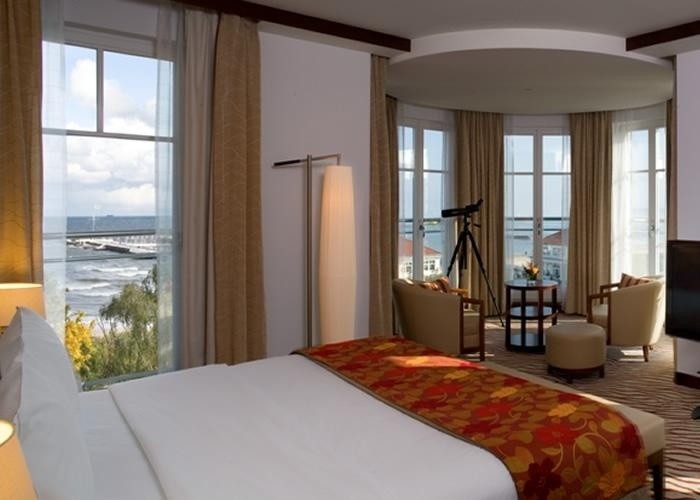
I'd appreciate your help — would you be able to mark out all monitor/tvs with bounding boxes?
[666,240,700,343]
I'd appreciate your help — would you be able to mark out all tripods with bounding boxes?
[446,217,504,328]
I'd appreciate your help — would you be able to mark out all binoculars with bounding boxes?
[441,198,483,217]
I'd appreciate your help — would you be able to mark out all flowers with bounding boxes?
[524,263,540,279]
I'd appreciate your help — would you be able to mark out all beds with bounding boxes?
[77,337,666,499]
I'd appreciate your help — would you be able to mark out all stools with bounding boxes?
[544,322,607,384]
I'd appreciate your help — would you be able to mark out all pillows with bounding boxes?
[422,276,453,296]
[620,274,656,291]
[3,312,92,500]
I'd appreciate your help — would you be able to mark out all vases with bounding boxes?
[527,274,542,284]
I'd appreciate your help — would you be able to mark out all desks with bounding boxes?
[504,279,560,354]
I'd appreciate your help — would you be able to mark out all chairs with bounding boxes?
[393,275,487,362]
[584,273,664,362]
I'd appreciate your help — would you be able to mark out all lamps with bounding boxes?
[1,278,46,325]
[275,150,360,352]
